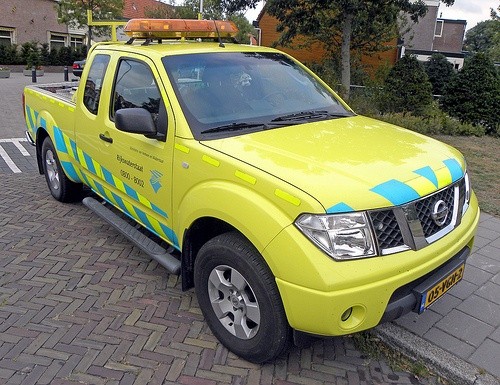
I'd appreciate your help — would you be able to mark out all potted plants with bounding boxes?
[23,53,44,76]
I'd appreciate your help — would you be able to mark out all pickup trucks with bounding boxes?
[20,16,482,366]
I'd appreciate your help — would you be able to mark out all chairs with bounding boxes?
[119,65,159,114]
[196,62,242,117]
